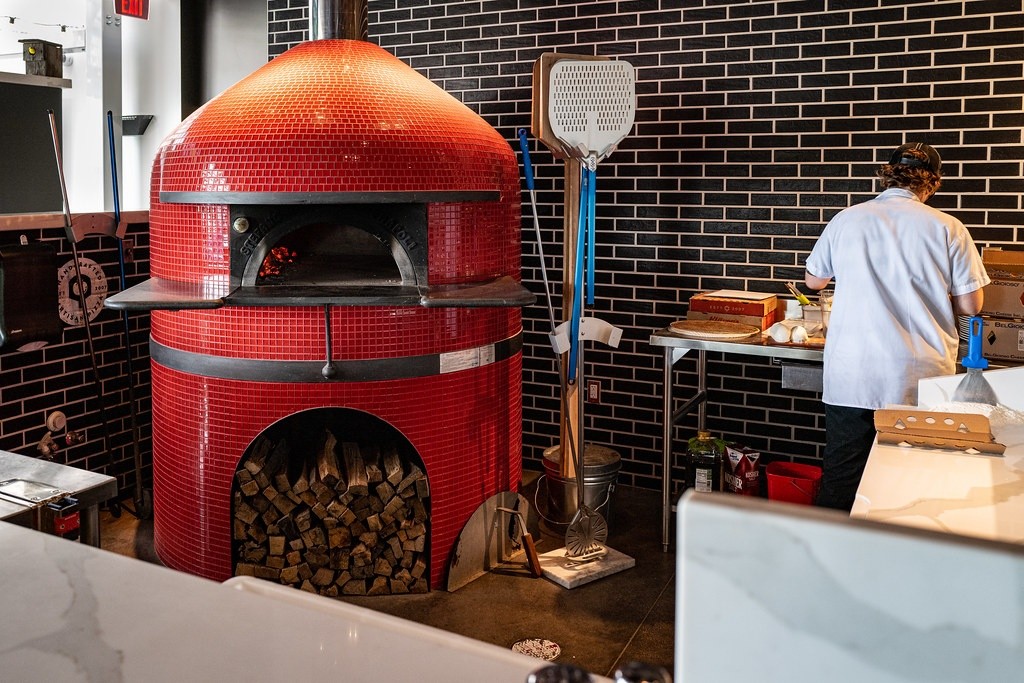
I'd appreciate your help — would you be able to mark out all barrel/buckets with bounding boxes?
[534,445,623,537]
[766,461,822,506]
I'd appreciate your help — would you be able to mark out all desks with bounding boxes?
[649,328,1024,573]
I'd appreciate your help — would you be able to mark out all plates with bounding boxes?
[669,319,758,341]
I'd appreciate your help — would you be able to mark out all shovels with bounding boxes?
[528,51,636,480]
[518,128,608,558]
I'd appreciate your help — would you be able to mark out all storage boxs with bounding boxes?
[973,247,1024,363]
[722,442,760,498]
[686,291,778,333]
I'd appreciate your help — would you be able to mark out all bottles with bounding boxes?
[684,430,721,493]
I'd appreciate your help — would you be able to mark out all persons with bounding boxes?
[803,140,992,515]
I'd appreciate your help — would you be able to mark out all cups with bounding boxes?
[817,289,834,338]
